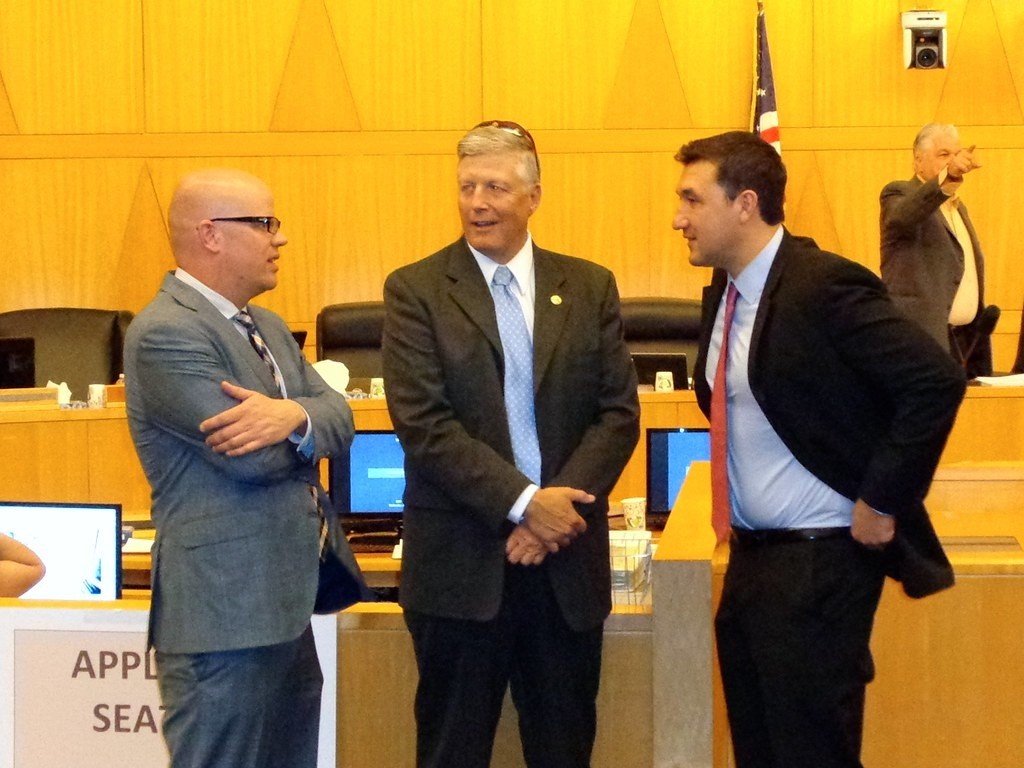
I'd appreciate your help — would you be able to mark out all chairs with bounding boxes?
[618,297,704,379]
[316,302,386,379]
[0,308,123,403]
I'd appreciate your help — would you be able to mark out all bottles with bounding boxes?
[115,374,124,386]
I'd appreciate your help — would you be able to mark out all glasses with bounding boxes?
[196,216,281,236]
[472,116,541,179]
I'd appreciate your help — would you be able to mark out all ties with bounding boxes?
[710,282,739,548]
[490,265,541,491]
[232,309,331,568]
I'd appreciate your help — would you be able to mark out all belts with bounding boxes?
[944,324,976,333]
[729,526,850,548]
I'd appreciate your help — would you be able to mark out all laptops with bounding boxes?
[328,428,407,551]
[0,502,123,600]
[0,337,36,390]
[290,330,307,350]
[644,427,713,529]
[628,352,688,390]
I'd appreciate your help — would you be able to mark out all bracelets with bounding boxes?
[947,173,962,182]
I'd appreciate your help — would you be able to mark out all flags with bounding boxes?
[749,11,781,154]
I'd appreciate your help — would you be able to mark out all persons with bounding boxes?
[382,120,640,768]
[880,121,992,378]
[0,534,46,599]
[671,130,967,768]
[123,169,368,768]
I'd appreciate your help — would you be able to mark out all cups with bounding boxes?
[88,384,106,408]
[655,371,674,393]
[370,377,385,398]
[621,497,647,531]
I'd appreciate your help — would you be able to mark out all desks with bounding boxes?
[0,379,1024,767]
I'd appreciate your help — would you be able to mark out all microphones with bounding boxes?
[963,305,1001,367]
